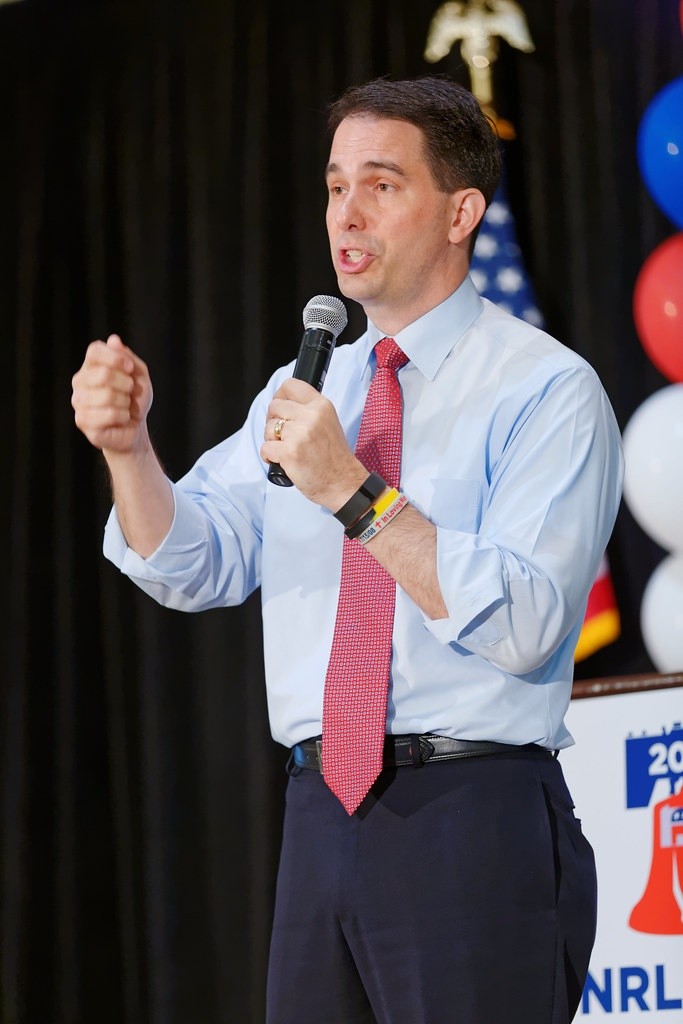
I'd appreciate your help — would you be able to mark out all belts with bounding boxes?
[296,735,543,772]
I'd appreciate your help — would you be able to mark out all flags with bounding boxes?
[471,202,621,664]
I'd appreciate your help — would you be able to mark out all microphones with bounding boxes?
[267,295,348,489]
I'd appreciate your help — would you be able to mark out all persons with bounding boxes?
[72,78,624,1023]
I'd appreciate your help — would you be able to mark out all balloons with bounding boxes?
[642,79,682,230]
[641,555,683,674]
[620,382,683,555]
[634,231,682,382]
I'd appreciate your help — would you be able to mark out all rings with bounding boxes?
[274,419,286,440]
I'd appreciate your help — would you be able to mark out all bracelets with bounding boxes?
[356,494,407,544]
[334,472,385,525]
[344,488,398,539]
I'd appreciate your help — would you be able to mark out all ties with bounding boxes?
[321,337,410,816]
